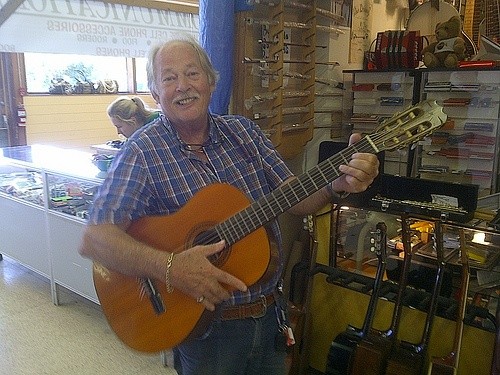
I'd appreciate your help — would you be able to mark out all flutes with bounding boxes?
[371,196,469,216]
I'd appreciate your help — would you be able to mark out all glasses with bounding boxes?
[168,112,224,151]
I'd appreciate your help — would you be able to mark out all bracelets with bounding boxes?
[327,182,350,198]
[166,252,174,295]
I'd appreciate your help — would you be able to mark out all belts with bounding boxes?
[214,287,283,321]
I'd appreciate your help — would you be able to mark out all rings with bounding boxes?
[197,295,205,302]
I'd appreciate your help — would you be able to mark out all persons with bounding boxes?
[79,36,380,375]
[92,97,164,160]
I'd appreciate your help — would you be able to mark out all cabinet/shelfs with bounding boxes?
[341,67,500,191]
[269,0,318,150]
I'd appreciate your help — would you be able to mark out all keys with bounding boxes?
[276,328,295,352]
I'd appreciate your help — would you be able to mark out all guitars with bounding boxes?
[324,222,387,375]
[93,99,448,355]
[348,212,414,375]
[382,220,447,375]
[427,228,471,375]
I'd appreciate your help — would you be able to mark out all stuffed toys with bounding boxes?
[420,15,465,69]
